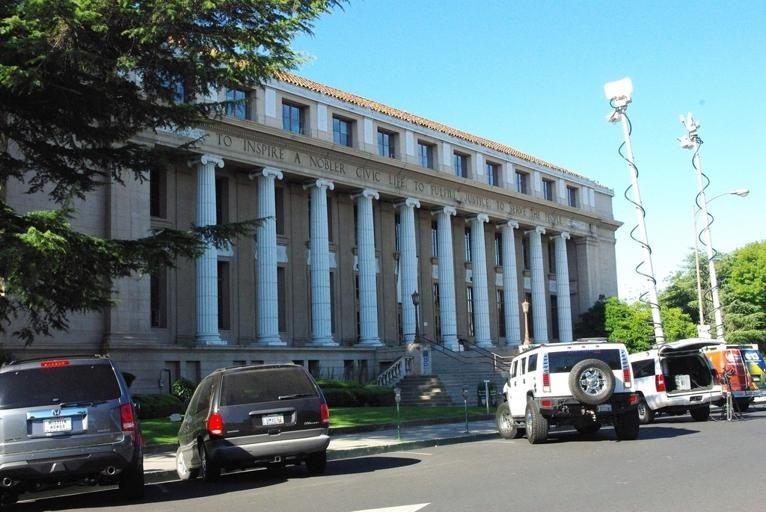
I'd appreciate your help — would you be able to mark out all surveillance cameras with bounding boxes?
[679,136,690,142]
[608,110,617,123]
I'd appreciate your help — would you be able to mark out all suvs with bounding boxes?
[702,342,766,412]
[495,335,641,444]
[1,350,147,509]
[169,360,331,487]
[628,336,724,425]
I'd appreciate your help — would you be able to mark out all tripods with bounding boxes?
[720,377,743,421]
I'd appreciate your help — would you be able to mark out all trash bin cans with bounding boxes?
[477,382,497,408]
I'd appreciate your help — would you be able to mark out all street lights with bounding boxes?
[521,298,532,344]
[411,290,421,343]
[605,75,665,348]
[691,187,751,338]
[678,111,728,338]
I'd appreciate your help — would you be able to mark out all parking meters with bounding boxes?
[393,386,401,441]
[462,384,470,433]
